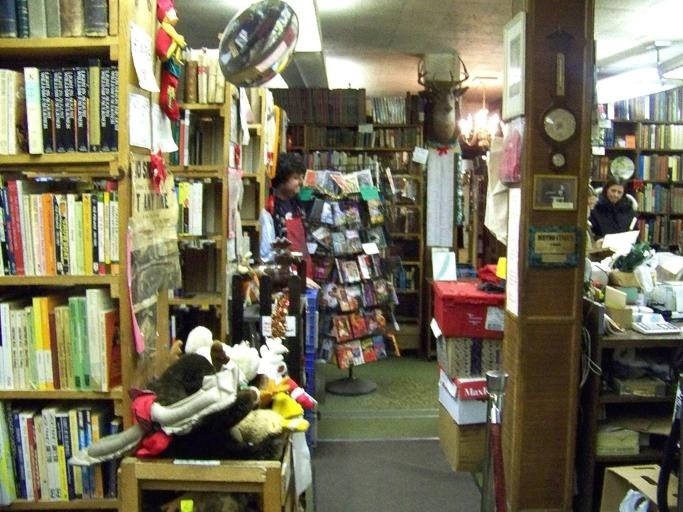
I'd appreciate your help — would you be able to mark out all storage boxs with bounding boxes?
[433,278,507,470]
[599,464,680,511]
[595,424,640,457]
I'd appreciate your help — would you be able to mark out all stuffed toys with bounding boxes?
[68,324,318,512]
[155,0,188,120]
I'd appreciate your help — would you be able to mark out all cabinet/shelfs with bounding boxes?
[301,168,401,397]
[1,0,169,512]
[231,260,307,387]
[118,428,293,512]
[169,47,242,341]
[242,81,266,232]
[269,89,424,349]
[572,296,683,511]
[591,87,683,254]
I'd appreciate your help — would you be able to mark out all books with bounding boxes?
[591,85,683,246]
[170,49,224,352]
[235,84,427,369]
[632,322,680,335]
[0,1,122,505]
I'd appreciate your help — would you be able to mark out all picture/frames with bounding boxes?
[502,11,526,122]
[533,174,578,210]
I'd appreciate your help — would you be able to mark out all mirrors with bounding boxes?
[219,0,299,87]
